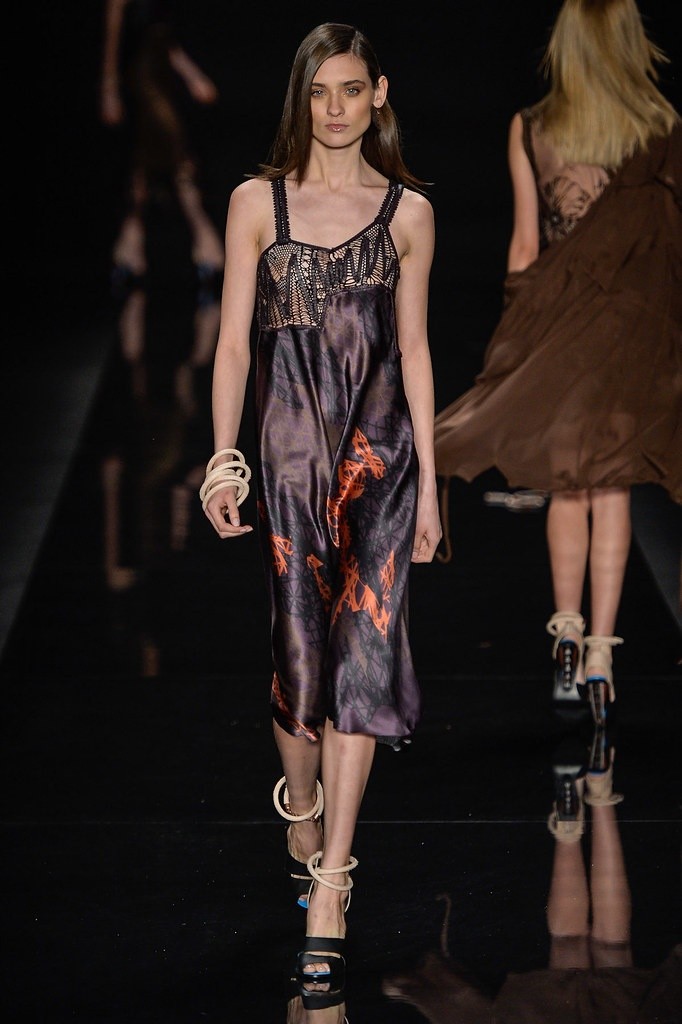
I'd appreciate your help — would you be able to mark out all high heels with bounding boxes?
[546,612,623,725]
[547,728,623,842]
[272,775,360,1008]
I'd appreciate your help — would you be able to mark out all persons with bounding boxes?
[433,0,682,719]
[101,282,219,683]
[199,21,442,1010]
[103,0,225,272]
[383,726,682,1024]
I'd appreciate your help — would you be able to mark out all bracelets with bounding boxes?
[198,448,251,514]
